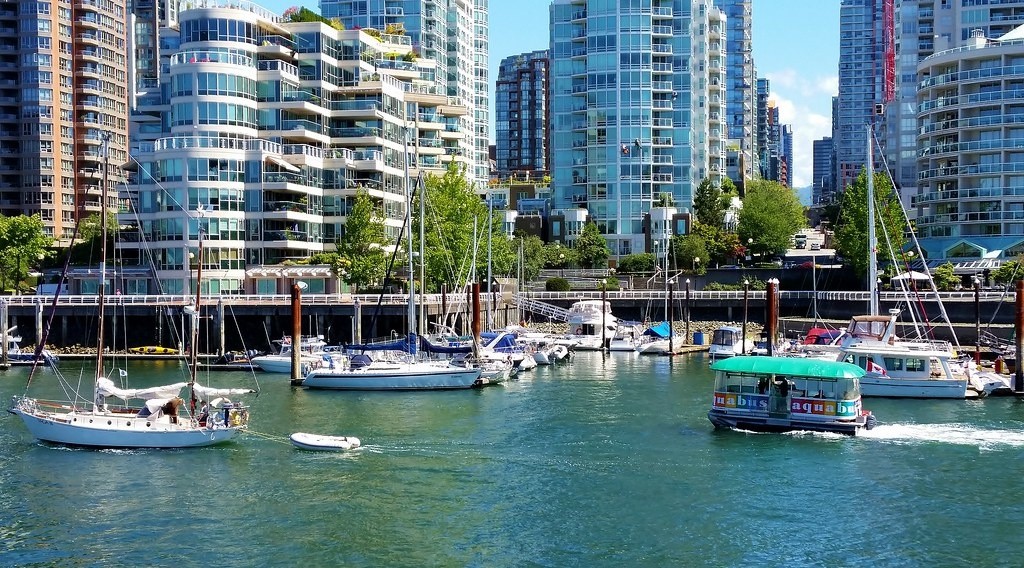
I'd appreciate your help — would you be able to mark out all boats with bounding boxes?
[128,346,179,353]
[707,355,876,438]
[835,124,968,399]
[707,327,745,360]
[0,333,58,365]
[566,298,617,350]
[290,432,362,451]
[609,318,647,350]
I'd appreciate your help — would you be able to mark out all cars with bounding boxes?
[812,242,822,251]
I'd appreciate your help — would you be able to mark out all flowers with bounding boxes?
[283,6,300,22]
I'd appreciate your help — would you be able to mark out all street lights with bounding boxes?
[558,253,565,280]
[693,257,701,291]
[908,250,914,291]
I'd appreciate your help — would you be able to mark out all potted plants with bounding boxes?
[278,228,301,240]
[309,97,320,105]
[370,128,378,136]
[268,175,305,185]
[335,150,342,158]
[277,202,302,213]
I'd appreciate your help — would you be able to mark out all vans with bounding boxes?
[789,233,808,249]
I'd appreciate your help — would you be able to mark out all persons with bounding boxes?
[634,140,641,151]
[620,143,631,154]
[776,376,790,413]
[758,374,772,396]
[198,400,210,427]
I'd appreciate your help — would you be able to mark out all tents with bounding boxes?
[890,270,935,292]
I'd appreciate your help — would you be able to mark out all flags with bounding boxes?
[866,360,887,375]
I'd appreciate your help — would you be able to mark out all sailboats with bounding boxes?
[3,131,260,449]
[635,193,687,355]
[250,167,579,392]
